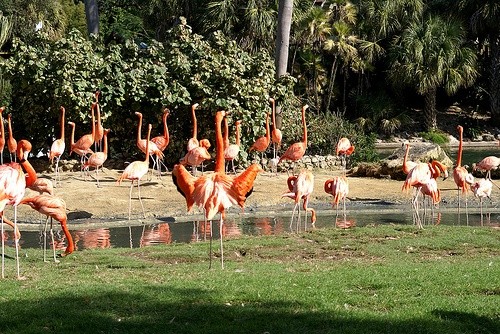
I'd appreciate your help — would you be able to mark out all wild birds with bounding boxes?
[135,108,171,182]
[116,124,152,221]
[0,140,75,281]
[281,171,317,234]
[68,90,111,189]
[452,125,500,229]
[324,174,350,229]
[49,105,65,190]
[271,97,281,176]
[402,141,448,230]
[335,136,356,177]
[278,103,310,176]
[0,105,18,165]
[171,102,270,271]
